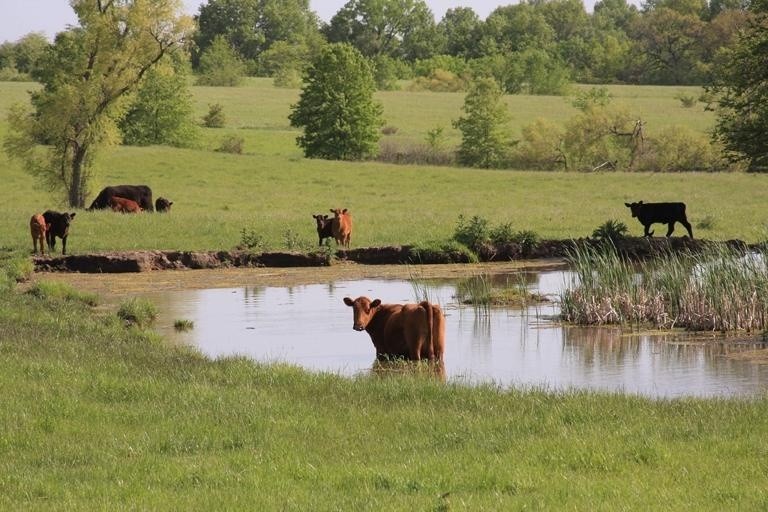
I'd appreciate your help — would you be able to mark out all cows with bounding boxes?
[42,210,76,256]
[312,214,334,246]
[85,185,155,216]
[329,208,353,249]
[624,200,693,239]
[155,197,174,213]
[343,295,445,368]
[29,213,52,256]
